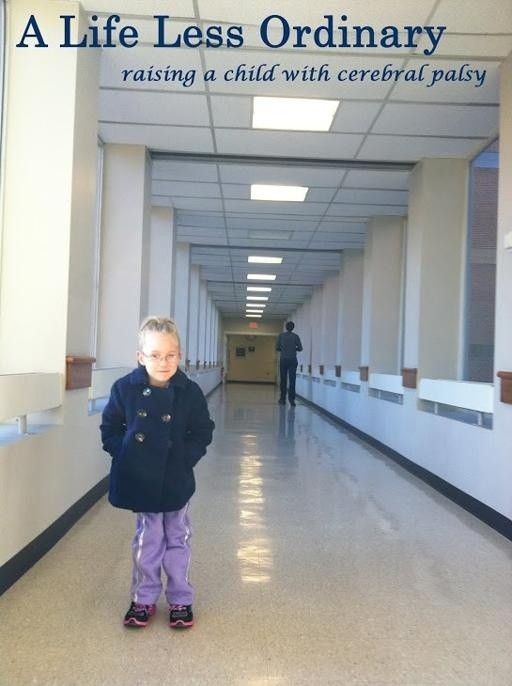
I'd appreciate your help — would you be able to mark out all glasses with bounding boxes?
[139,351,182,365]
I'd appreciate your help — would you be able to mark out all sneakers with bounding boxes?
[169,604,194,628]
[277,400,286,405]
[124,603,157,625]
[288,397,296,405]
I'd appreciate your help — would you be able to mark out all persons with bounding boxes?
[98,314,216,629]
[273,319,304,405]
